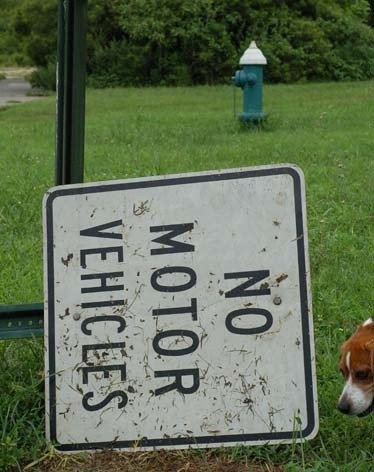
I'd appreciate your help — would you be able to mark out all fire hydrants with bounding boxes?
[231,41,267,122]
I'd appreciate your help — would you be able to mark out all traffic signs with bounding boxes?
[43,163,319,456]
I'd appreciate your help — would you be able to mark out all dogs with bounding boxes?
[337,315,374,418]
